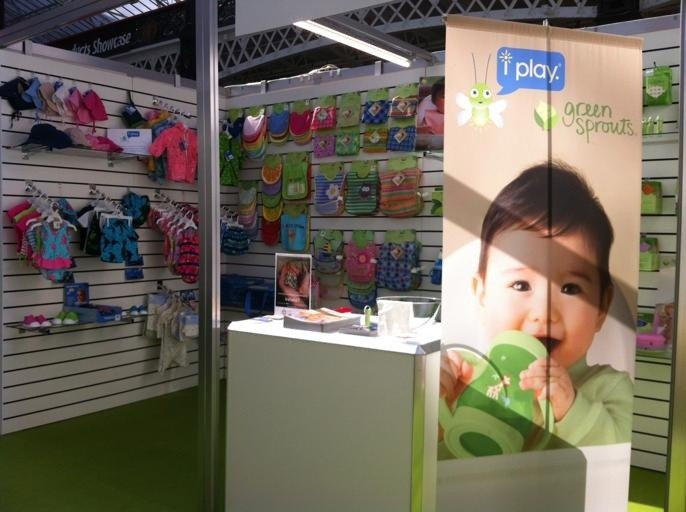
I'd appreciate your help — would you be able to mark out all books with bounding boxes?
[284,306,361,332]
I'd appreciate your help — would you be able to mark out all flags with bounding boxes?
[432,13,647,512]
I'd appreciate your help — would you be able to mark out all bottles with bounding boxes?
[642,115,664,134]
[443,328,557,459]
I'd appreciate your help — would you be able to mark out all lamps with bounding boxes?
[293,15,438,69]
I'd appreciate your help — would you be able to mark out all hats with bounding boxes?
[17,124,124,152]
[0,76,109,123]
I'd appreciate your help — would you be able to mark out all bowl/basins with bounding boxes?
[375,295,442,329]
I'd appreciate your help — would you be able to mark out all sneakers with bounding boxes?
[35,314,52,328]
[51,311,68,326]
[127,304,139,316]
[138,304,148,316]
[63,312,79,326]
[22,314,41,329]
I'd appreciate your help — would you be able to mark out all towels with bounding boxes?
[375,299,416,343]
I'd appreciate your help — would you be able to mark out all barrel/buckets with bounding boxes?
[376,296,441,322]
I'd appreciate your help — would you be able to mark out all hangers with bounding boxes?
[23,180,244,236]
[159,100,183,121]
[165,292,188,313]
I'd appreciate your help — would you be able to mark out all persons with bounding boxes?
[439,156,635,456]
[279,262,310,309]
